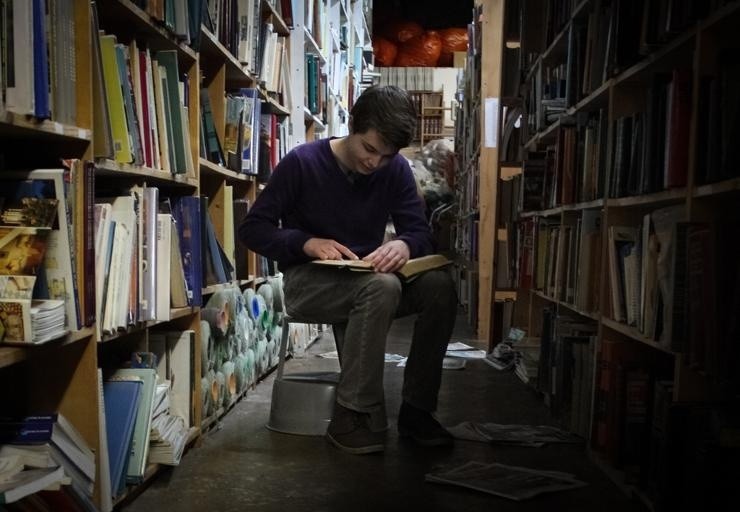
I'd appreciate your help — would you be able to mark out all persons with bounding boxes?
[237,85,460,473]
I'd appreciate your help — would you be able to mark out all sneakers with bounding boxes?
[399,408,457,447]
[328,411,386,455]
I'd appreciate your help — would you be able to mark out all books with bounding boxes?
[310,254,454,284]
[453,1,739,512]
[2,0,351,511]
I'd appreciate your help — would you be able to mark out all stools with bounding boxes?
[267,319,346,438]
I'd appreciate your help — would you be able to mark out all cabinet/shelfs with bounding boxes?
[513,2,740,510]
[0,1,368,511]
[447,0,521,339]
[404,87,446,144]
